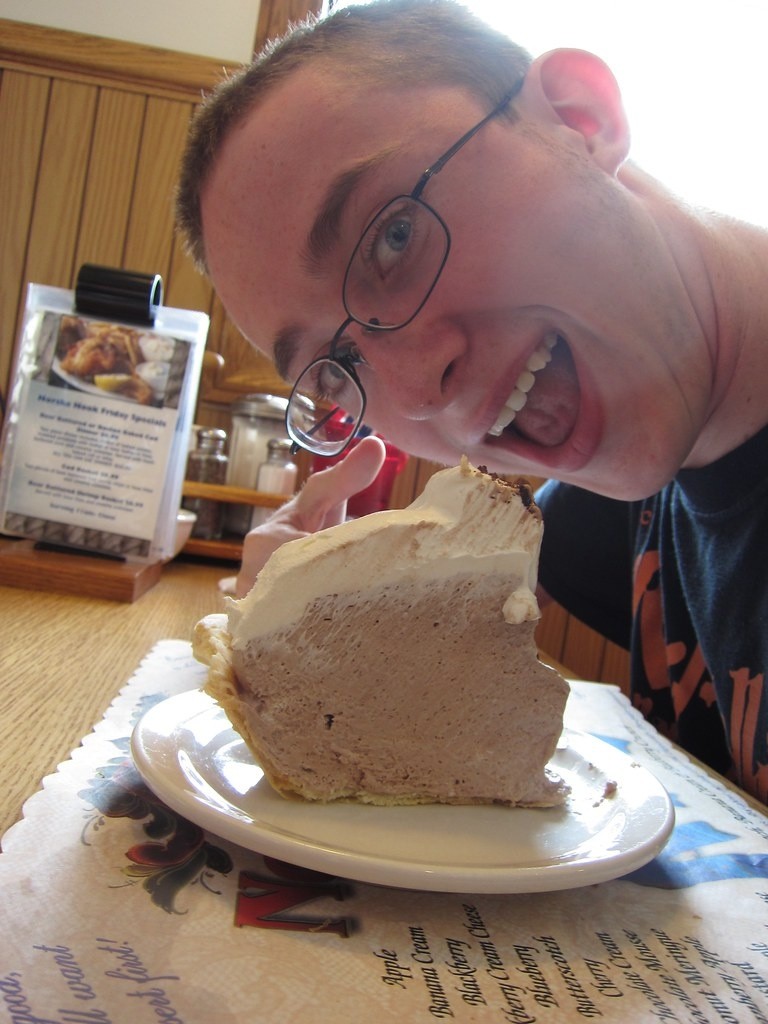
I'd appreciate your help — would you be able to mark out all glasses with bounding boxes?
[283,74,525,459]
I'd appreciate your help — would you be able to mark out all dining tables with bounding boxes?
[1,561,768,1024]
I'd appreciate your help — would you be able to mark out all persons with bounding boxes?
[174,0,768,806]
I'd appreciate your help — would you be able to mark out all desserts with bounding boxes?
[187,455,574,810]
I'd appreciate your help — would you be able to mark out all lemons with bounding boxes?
[94,372,130,390]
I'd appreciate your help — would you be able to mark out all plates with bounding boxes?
[130,690,675,893]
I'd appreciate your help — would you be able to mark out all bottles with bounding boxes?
[226,394,289,535]
[249,438,298,529]
[182,427,229,537]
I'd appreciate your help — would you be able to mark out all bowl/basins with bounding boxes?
[161,510,197,566]
